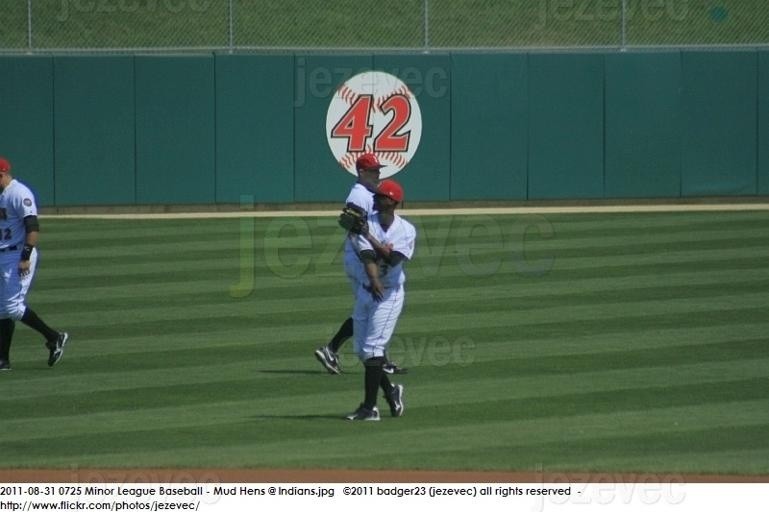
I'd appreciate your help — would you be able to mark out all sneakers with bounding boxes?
[0,359,12,371]
[346,402,380,422]
[382,362,401,374]
[45,331,69,367]
[314,345,343,375]
[385,384,404,418]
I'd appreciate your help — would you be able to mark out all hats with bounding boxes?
[356,154,387,171]
[367,182,404,204]
[0,158,10,171]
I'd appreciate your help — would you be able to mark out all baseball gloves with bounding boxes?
[338,202,369,235]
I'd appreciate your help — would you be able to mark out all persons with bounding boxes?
[0,157,69,371]
[346,179,416,420]
[314,153,408,374]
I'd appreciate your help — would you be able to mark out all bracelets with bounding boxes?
[21,243,33,260]
